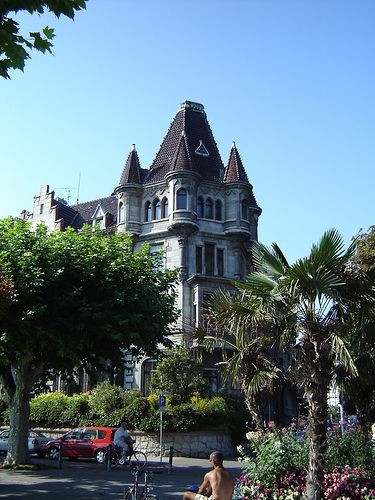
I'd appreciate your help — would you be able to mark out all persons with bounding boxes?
[183,450,233,500]
[114,423,136,460]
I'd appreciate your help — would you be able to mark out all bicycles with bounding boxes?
[123,464,160,500]
[104,446,148,469]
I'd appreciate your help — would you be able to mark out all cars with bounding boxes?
[0,429,54,457]
[45,426,134,463]
